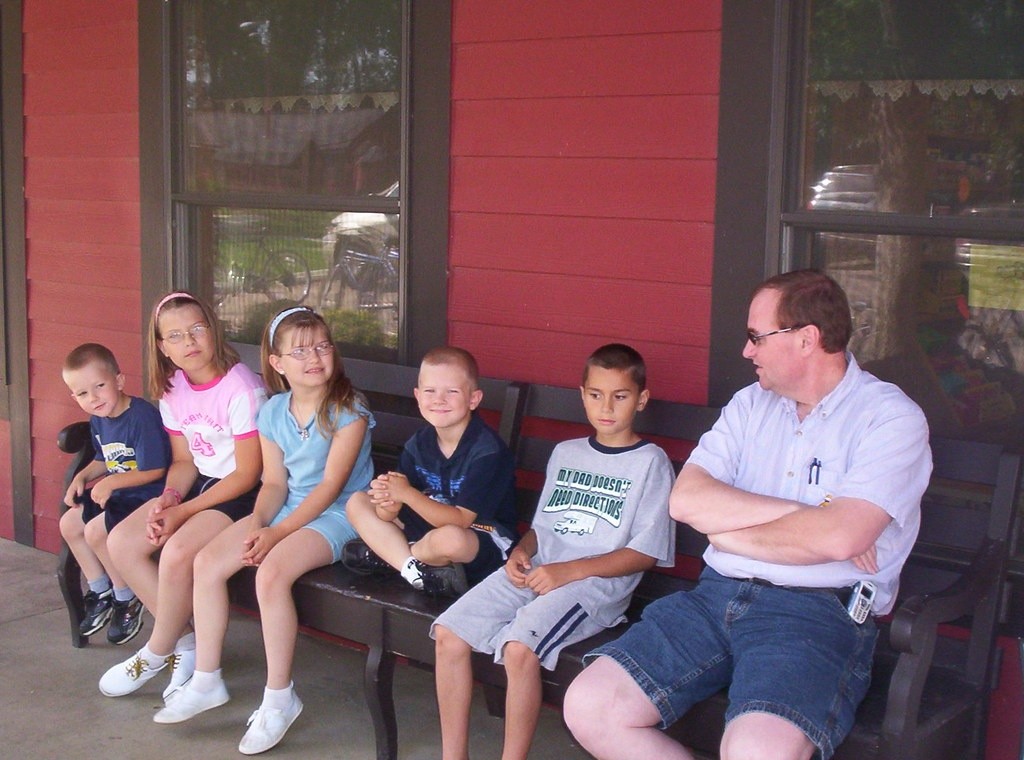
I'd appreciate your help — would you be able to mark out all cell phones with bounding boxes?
[847,580,877,624]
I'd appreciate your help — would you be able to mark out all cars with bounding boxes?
[320,180,400,288]
[954,204,1024,270]
[804,164,886,259]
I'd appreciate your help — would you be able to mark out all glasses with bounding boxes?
[280,343,335,362]
[160,324,212,344]
[746,325,808,346]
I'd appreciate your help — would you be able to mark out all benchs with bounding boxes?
[54,345,1022,760]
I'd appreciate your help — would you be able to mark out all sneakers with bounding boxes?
[106,593,144,645]
[153,676,230,722]
[99,649,174,697]
[413,557,469,602]
[340,538,424,573]
[161,648,198,702]
[78,584,115,637]
[240,689,304,754]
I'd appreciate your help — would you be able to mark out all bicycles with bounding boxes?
[317,230,400,337]
[210,215,314,311]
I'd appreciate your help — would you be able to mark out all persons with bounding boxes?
[429,343,676,760]
[563,269,933,760]
[99,291,267,702]
[154,306,374,756]
[62,344,172,643]
[340,347,514,603]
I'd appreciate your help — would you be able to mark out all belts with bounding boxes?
[733,572,854,595]
